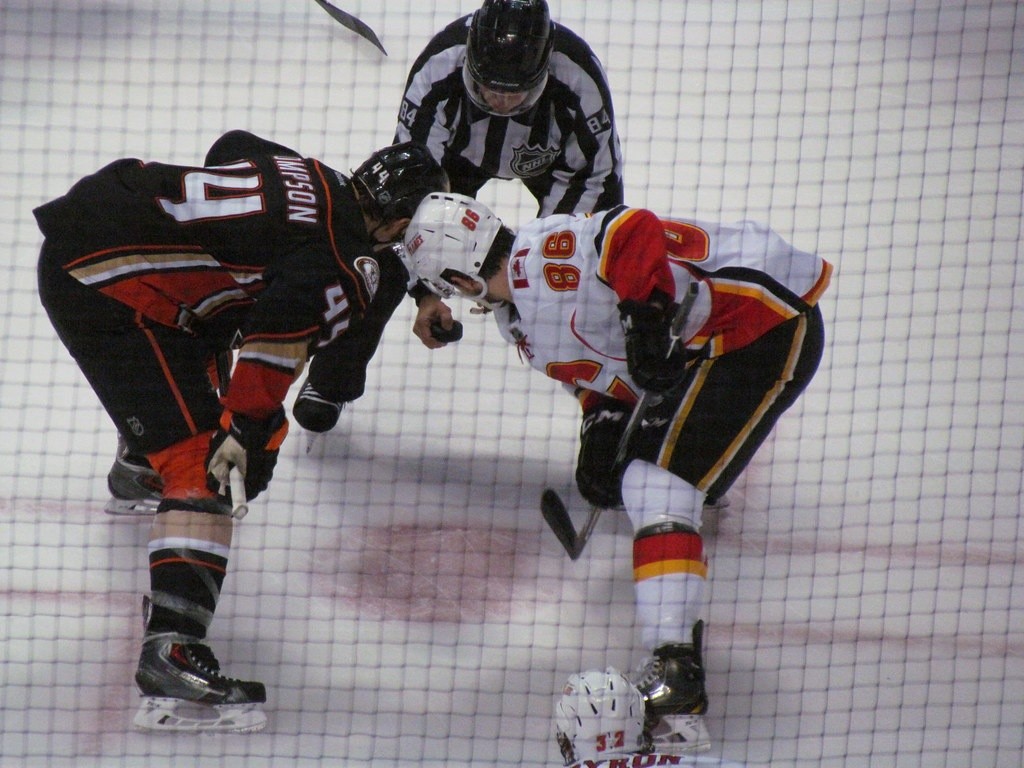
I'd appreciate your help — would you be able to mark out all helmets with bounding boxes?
[404,191,504,299]
[555,667,648,759]
[355,141,452,218]
[466,0,553,91]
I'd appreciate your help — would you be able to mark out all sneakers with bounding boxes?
[633,618,712,755]
[103,431,167,517]
[292,375,345,453]
[135,595,268,732]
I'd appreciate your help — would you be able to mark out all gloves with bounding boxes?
[617,287,686,393]
[204,407,288,515]
[577,405,631,510]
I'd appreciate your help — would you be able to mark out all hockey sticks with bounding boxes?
[315,0,388,56]
[215,349,249,521]
[540,281,699,561]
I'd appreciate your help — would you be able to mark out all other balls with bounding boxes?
[431,319,463,343]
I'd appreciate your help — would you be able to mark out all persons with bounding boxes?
[33,128,451,735]
[555,666,698,768]
[403,191,833,754]
[293,0,645,510]
